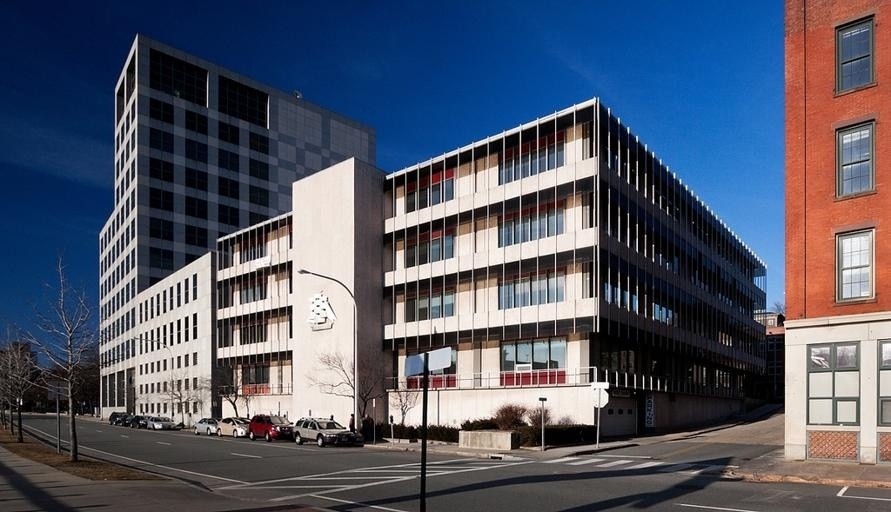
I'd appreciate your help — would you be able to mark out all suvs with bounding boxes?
[292,418,359,447]
[246,412,294,441]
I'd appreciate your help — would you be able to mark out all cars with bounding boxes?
[193,416,222,436]
[107,411,133,427]
[131,415,150,429]
[216,417,253,438]
[146,414,175,430]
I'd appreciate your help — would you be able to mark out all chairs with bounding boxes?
[312,422,336,429]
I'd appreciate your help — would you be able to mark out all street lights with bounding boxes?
[297,267,357,430]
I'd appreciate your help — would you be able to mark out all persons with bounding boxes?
[349,414,355,432]
[330,415,334,420]
